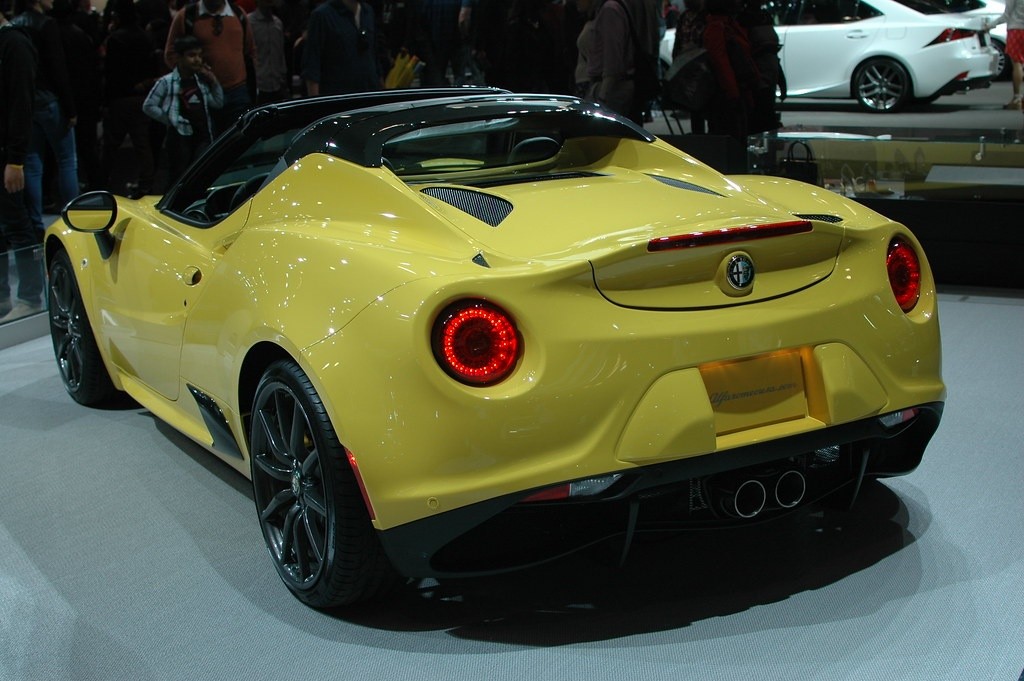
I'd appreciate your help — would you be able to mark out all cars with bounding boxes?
[43,82,948,616]
[654,0,1014,113]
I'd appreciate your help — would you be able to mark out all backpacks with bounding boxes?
[497,10,538,79]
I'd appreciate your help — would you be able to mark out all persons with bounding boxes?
[983,0,1024,110]
[0,0,786,326]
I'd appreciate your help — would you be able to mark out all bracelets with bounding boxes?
[7,164,24,169]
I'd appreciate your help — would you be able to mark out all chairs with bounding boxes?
[507,135,560,167]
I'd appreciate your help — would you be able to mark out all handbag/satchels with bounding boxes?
[720,20,754,76]
[677,58,719,112]
[634,51,675,98]
[148,124,167,150]
[779,140,825,188]
[451,43,490,87]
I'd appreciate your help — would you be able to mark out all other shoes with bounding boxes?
[44,180,59,208]
[1003,97,1024,110]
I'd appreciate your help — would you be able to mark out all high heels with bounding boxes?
[840,164,895,196]
